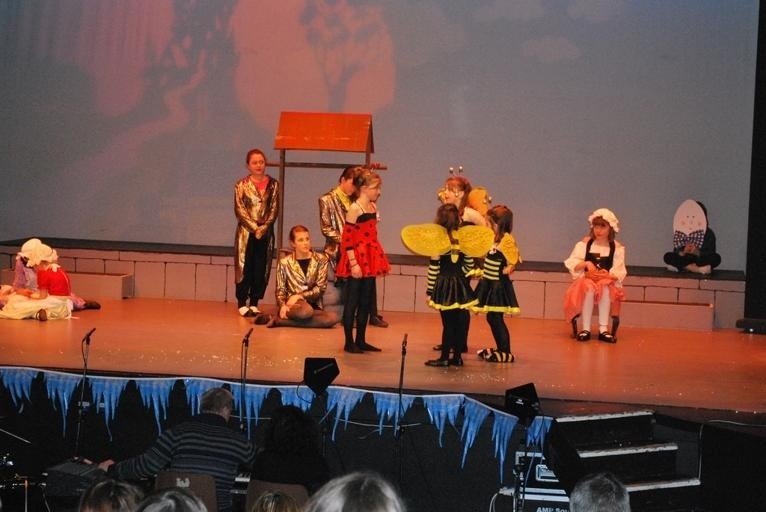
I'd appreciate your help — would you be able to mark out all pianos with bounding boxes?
[231,472,252,494]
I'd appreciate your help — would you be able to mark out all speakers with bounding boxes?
[503,383,541,409]
[301,355,340,395]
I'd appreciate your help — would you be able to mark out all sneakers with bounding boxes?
[477,348,514,362]
[704,265,711,274]
[249,306,261,314]
[36,309,47,321]
[370,317,388,327]
[239,306,249,316]
[425,361,449,366]
[665,264,678,272]
[449,359,463,366]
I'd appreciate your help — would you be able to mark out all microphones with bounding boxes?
[86,327,96,337]
[244,328,254,341]
[402,334,408,347]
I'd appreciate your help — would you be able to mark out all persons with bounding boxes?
[233,149,280,318]
[250,488,302,511]
[135,484,210,511]
[565,205,628,341]
[98,386,258,510]
[337,167,388,355]
[302,468,408,512]
[477,206,520,361]
[569,472,631,511]
[0,239,74,322]
[252,226,339,329]
[663,198,725,275]
[0,236,102,310]
[317,169,389,327]
[428,204,469,370]
[79,479,143,511]
[433,174,481,353]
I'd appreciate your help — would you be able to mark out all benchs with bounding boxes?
[1,266,133,302]
[619,299,715,332]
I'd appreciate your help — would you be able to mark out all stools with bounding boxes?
[570,309,620,342]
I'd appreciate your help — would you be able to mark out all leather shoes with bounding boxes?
[577,330,590,341]
[84,300,100,309]
[599,330,617,343]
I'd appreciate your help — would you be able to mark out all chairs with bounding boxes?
[244,480,309,512]
[156,469,218,512]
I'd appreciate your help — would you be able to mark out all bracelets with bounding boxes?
[348,263,360,266]
[347,257,357,260]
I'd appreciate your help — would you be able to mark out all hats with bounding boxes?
[19,238,57,263]
[588,208,620,233]
